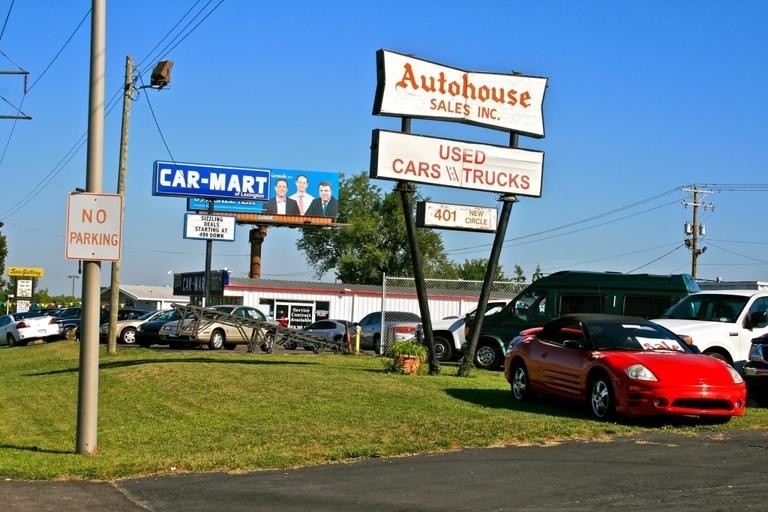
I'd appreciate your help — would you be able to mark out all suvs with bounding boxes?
[624,287,768,365]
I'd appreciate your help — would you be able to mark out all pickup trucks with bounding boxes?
[426,299,523,362]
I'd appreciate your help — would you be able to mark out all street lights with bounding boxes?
[109,54,174,353]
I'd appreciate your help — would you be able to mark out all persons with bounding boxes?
[304,182,339,216]
[261,178,300,216]
[289,175,316,216]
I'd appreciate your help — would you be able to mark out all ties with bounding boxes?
[323,201,326,215]
[298,195,305,216]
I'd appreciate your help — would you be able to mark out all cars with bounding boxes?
[285,318,355,353]
[162,303,280,352]
[100,307,178,347]
[0,311,59,347]
[348,311,424,355]
[35,306,105,339]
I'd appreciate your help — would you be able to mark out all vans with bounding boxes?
[466,270,702,368]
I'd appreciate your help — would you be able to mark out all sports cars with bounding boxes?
[504,312,748,422]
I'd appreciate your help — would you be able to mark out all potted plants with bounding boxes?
[383,340,430,375]
